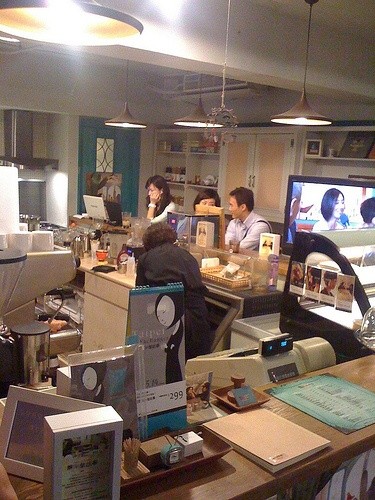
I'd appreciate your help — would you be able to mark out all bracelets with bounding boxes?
[147,203,156,208]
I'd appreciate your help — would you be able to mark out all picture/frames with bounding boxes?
[0,384,105,483]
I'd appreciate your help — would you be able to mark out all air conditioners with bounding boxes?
[182,71,250,95]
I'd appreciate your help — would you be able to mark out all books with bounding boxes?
[203,405,330,474]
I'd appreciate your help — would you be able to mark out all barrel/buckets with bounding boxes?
[10,321,51,388]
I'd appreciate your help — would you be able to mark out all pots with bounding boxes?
[19,213,40,232]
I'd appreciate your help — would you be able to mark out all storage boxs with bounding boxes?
[44,405,124,500]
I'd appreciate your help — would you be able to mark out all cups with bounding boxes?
[228,240,240,253]
[54,210,152,278]
[195,176,201,184]
[123,452,138,471]
[251,259,267,292]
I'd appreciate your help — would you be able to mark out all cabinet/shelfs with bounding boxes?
[154,128,297,224]
[299,123,375,178]
[36,265,132,354]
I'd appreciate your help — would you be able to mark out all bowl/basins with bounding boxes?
[96,250,109,261]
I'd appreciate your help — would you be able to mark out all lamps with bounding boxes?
[0,0,143,47]
[105,1,333,128]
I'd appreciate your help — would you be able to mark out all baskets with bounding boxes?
[199,264,250,288]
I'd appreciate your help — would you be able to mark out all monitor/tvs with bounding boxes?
[281,175,375,256]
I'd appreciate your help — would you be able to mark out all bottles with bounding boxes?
[118,243,127,272]
[165,165,185,183]
[266,254,280,290]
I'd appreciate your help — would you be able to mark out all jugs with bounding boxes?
[102,228,128,259]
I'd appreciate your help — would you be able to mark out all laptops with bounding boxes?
[83,195,116,223]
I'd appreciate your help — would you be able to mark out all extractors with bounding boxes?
[1,109,58,170]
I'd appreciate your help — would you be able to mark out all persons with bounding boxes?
[290,264,354,299]
[186,386,196,399]
[131,221,211,360]
[144,174,272,254]
[199,382,209,404]
[287,181,375,243]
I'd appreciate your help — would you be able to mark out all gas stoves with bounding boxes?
[40,221,67,232]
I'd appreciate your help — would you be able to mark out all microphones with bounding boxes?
[340,214,349,228]
